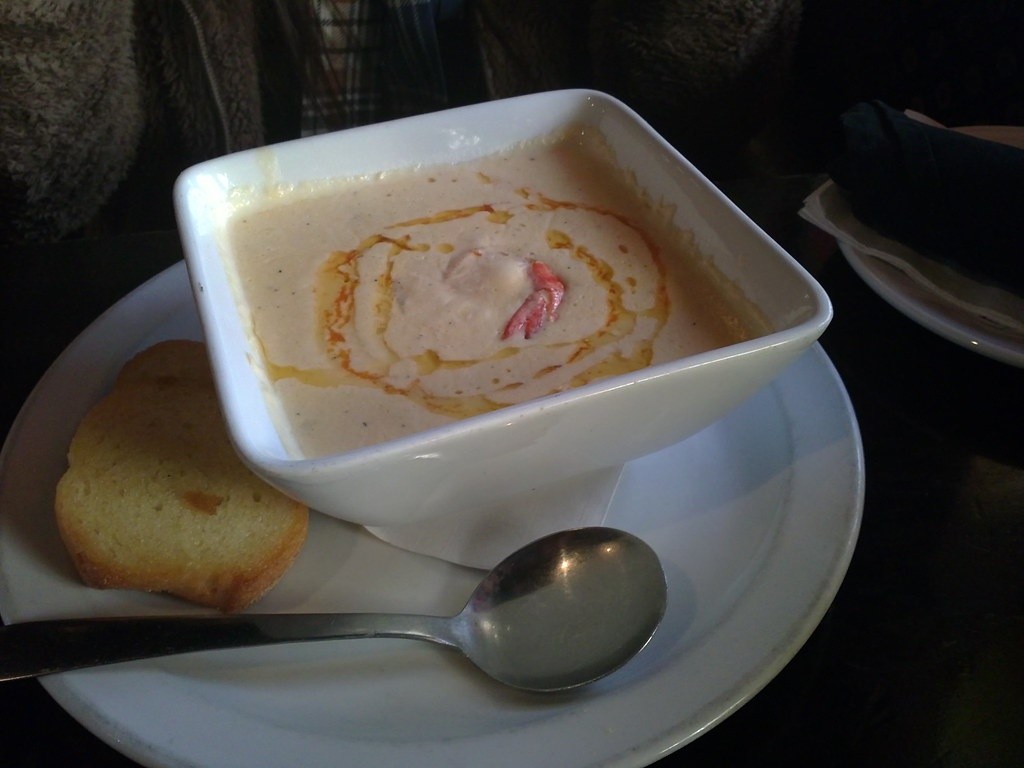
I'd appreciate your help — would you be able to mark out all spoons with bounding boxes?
[0,525,669,691]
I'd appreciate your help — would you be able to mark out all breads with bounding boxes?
[51,337,308,611]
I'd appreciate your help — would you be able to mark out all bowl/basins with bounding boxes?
[171,87,835,525]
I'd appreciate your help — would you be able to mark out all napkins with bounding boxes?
[794,107,1024,338]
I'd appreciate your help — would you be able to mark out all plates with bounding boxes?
[0,257,866,768]
[841,125,1024,367]
